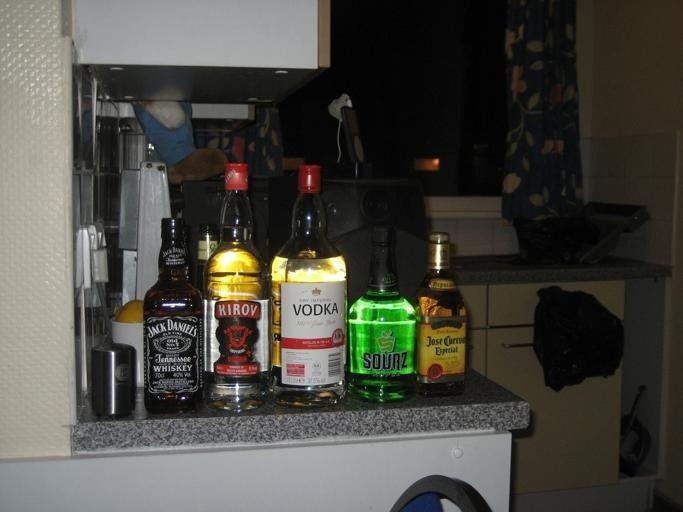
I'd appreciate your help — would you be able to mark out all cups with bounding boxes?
[90,342,136,418]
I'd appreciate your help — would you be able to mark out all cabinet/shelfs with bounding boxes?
[460,285,624,489]
[70,0,334,105]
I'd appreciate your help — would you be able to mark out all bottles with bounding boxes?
[420,233,472,394]
[282,164,346,405]
[351,230,416,400]
[207,163,275,410]
[150,216,197,409]
